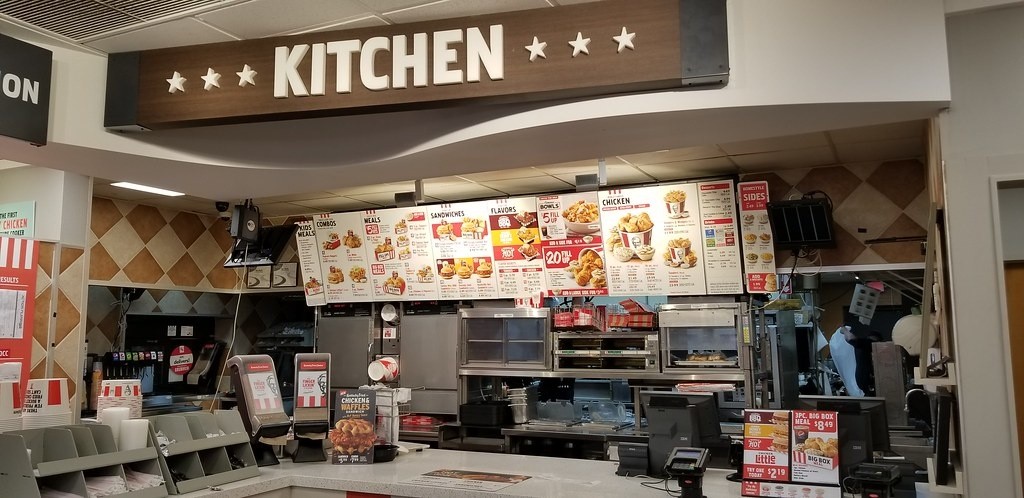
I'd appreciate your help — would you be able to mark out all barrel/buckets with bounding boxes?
[666,198,684,220]
[619,228,655,259]
[668,244,690,267]
[22,378,73,430]
[0,380,23,433]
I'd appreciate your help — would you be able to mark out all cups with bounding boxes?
[380,305,401,326]
[542,226,548,237]
[91,360,103,411]
[792,425,811,445]
[367,357,399,382]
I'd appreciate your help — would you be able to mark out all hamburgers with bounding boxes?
[441,265,491,279]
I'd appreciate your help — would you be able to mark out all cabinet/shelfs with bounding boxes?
[0,424,169,497]
[144,409,260,494]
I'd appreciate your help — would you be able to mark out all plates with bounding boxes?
[680,264,690,268]
[665,261,671,265]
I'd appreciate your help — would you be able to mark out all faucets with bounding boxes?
[902,388,936,446]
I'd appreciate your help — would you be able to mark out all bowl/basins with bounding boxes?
[561,213,601,235]
[96,379,144,422]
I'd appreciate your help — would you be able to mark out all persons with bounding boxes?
[840,324,876,397]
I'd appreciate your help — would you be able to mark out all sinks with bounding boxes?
[889,436,934,470]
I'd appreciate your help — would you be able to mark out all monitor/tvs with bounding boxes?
[766,198,836,249]
[639,391,722,436]
[798,395,891,479]
[223,224,300,268]
